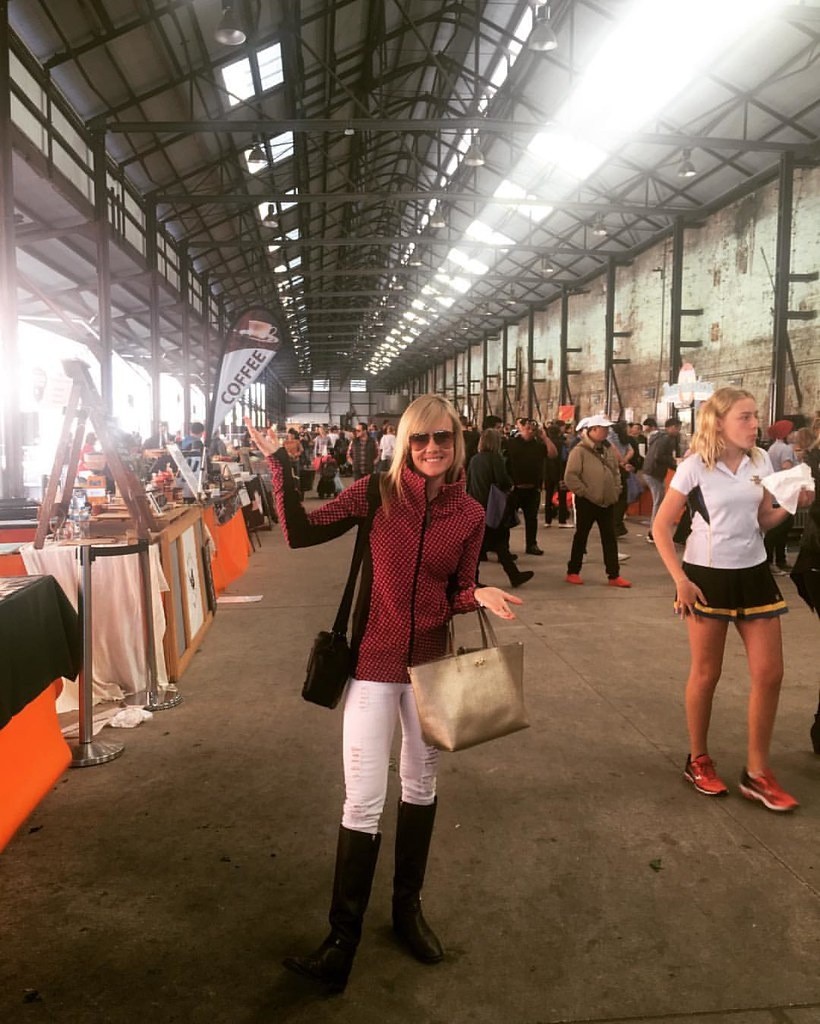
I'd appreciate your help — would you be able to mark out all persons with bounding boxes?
[181,421,204,472]
[175,430,182,442]
[648,387,814,812]
[207,427,227,458]
[244,391,523,998]
[266,416,396,498]
[159,421,175,450]
[458,414,691,588]
[764,411,820,754]
[77,431,98,481]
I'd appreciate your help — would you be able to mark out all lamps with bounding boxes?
[216,0,697,358]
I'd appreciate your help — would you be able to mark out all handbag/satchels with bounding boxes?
[626,471,643,504]
[300,631,352,710]
[484,485,509,530]
[406,586,531,752]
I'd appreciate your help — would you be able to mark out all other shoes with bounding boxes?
[525,545,543,555]
[770,563,793,576]
[544,523,551,528]
[618,553,631,561]
[498,553,518,562]
[559,523,574,528]
[511,571,534,588]
[646,534,653,542]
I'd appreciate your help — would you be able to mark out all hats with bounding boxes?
[588,415,616,430]
[665,418,681,427]
[766,420,793,440]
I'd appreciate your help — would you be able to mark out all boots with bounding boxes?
[392,794,444,965]
[282,821,381,993]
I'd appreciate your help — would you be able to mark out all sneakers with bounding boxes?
[737,766,800,811]
[608,577,632,588]
[566,574,583,584]
[684,753,731,797]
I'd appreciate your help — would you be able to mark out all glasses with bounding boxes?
[409,430,456,451]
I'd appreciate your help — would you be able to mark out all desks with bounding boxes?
[0,460,269,847]
